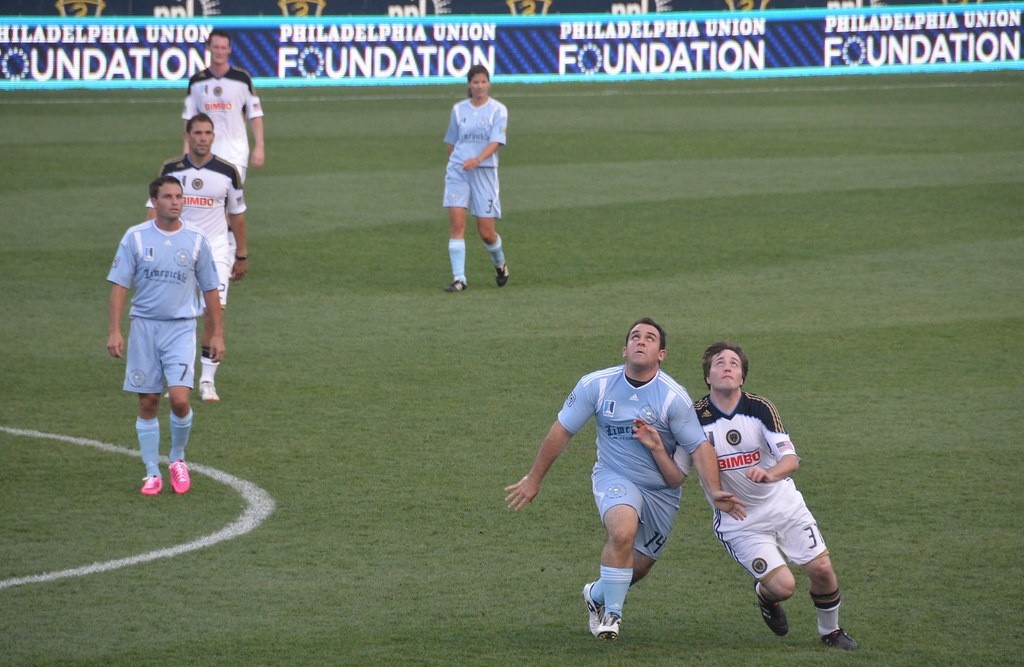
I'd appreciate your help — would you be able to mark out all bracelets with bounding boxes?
[475,158,481,164]
[236,255,248,260]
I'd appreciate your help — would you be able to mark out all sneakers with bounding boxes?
[446,280,467,293]
[821,627,857,651]
[583,581,605,639]
[199,380,220,403]
[495,262,509,287]
[141,474,163,496]
[754,580,788,637]
[169,457,190,494]
[597,612,622,641]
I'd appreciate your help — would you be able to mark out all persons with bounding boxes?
[107,176,225,495]
[146,113,248,402]
[633,342,859,651]
[181,28,264,278]
[504,318,747,642]
[443,65,509,292]
